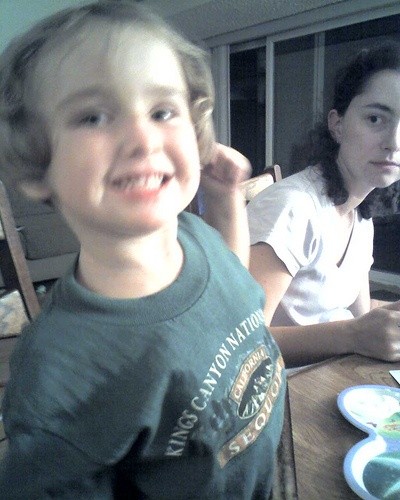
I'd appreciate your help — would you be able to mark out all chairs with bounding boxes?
[0,180,80,324]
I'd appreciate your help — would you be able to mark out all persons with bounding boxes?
[244,45,400,366]
[0,0,287,499]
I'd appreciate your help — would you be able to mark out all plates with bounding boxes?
[337,385,400,500]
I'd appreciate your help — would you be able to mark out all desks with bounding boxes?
[271,353,400,500]
